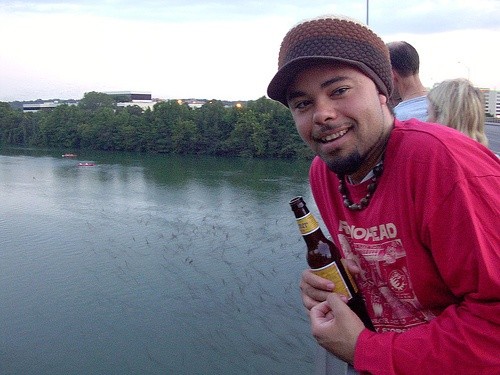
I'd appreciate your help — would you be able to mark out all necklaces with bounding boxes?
[337,164,382,211]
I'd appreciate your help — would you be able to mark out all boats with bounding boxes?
[76,164,96,168]
[64,153,77,158]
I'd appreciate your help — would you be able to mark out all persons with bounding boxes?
[424,79,488,146]
[385,40,429,122]
[267,13,500,375]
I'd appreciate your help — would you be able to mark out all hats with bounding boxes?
[267,18,394,108]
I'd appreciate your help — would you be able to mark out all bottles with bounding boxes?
[288,196,376,332]
